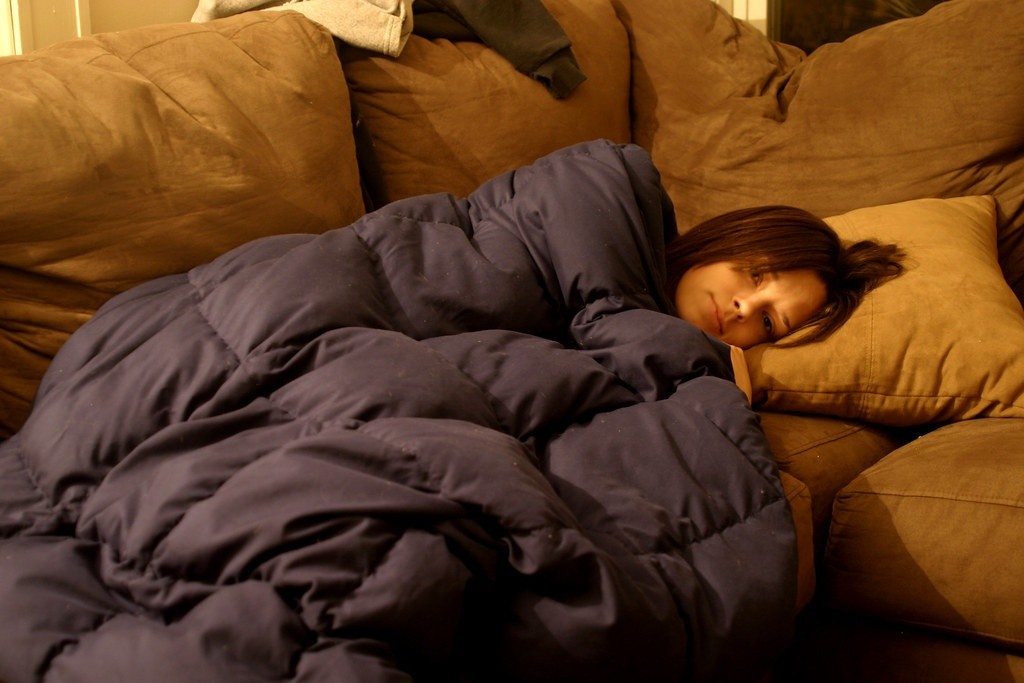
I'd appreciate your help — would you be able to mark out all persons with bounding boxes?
[658,203,905,412]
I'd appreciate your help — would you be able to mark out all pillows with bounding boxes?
[744,194,1024,425]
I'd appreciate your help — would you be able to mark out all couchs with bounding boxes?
[0,0,1024,683]
